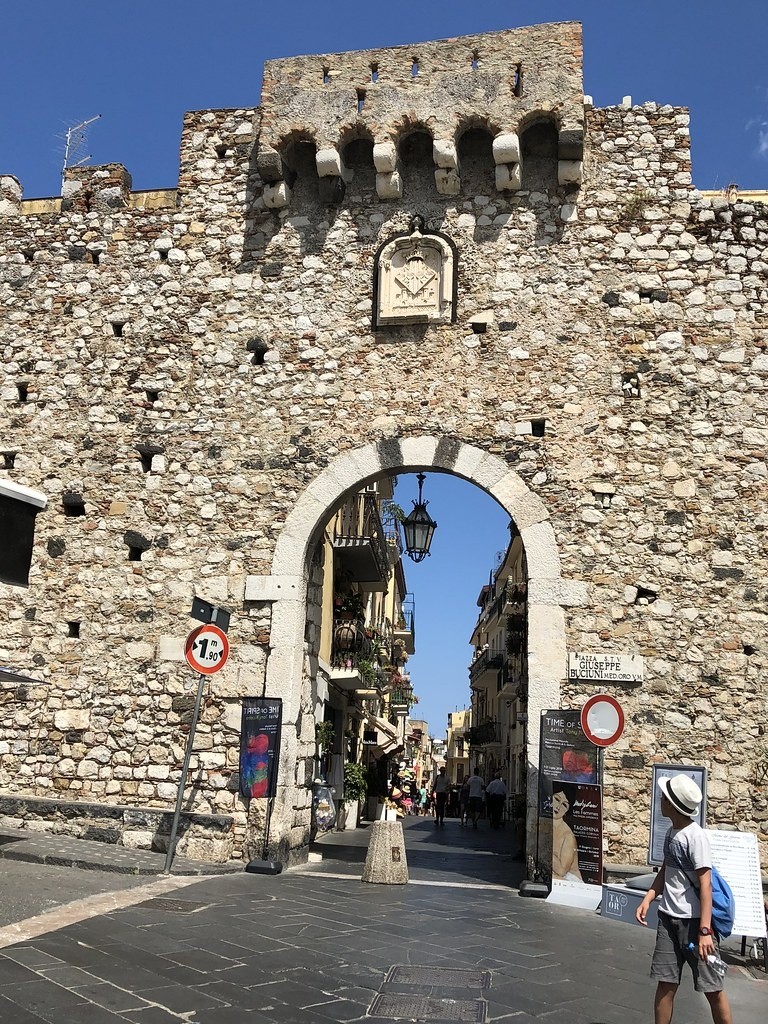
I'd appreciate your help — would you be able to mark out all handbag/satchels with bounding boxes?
[692,865,735,938]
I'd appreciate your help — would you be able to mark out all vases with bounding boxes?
[339,610,354,621]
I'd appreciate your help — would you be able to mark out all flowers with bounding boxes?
[333,589,368,617]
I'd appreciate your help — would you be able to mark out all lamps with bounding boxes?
[400,472,439,564]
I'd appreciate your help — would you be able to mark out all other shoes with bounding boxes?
[459,822,463,826]
[473,822,478,829]
[434,820,439,825]
[464,822,467,827]
[440,821,444,826]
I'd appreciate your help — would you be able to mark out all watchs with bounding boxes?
[700,925,713,936]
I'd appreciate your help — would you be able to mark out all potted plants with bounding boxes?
[340,761,368,831]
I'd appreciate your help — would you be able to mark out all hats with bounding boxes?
[439,767,446,771]
[403,785,410,793]
[658,776,703,817]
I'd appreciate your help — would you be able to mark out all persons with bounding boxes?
[413,766,508,830]
[635,775,733,1024]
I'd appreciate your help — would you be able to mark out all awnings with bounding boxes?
[372,718,405,761]
